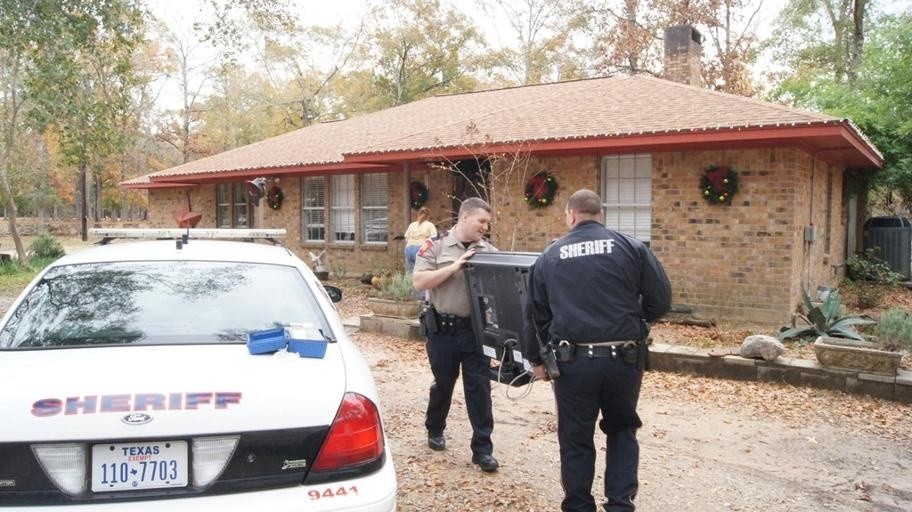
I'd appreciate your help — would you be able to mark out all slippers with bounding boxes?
[428,430,446,451]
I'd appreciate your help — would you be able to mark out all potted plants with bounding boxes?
[370,267,424,321]
[814,307,910,375]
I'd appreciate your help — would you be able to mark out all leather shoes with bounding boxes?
[470,452,498,471]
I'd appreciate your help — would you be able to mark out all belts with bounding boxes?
[455,318,471,327]
[573,344,622,359]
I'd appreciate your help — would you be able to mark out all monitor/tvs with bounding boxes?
[458,251,544,387]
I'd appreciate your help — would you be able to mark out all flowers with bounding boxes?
[266,186,284,209]
[521,171,558,209]
[698,164,739,207]
[410,182,428,209]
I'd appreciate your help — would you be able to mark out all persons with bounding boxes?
[410,197,510,472]
[522,187,670,512]
[404,205,436,299]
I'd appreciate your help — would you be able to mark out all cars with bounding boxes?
[0,227,399,511]
